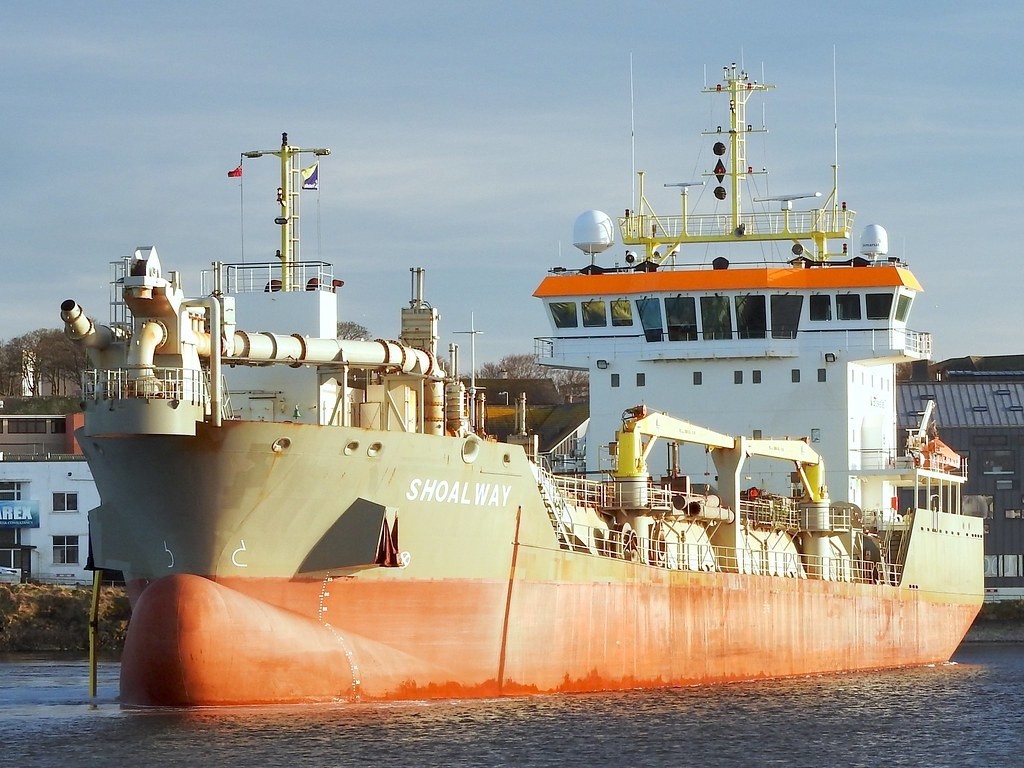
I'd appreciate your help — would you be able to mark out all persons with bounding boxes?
[928,423,938,440]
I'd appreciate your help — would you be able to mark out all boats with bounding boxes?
[61,38,987,710]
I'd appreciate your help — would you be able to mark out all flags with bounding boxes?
[228,164,242,178]
[300,161,319,190]
